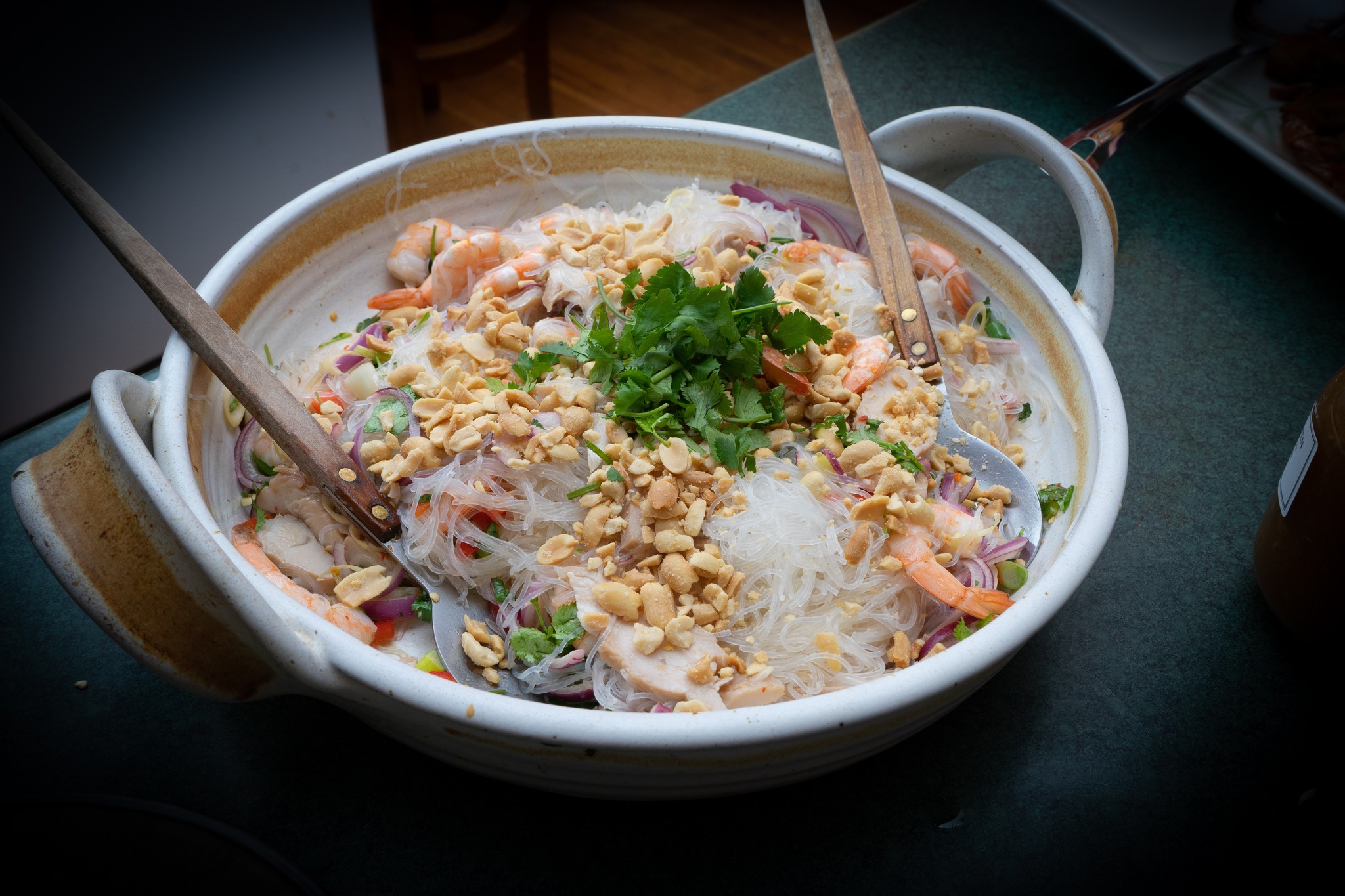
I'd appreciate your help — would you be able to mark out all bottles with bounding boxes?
[1252,366,1345,636]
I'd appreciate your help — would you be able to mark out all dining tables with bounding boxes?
[0,0,1345,896]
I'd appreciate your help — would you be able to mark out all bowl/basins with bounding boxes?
[13,102,1120,804]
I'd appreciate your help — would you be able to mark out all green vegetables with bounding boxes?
[496,255,929,482]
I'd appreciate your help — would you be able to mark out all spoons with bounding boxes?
[1,106,554,705]
[802,0,1042,563]
